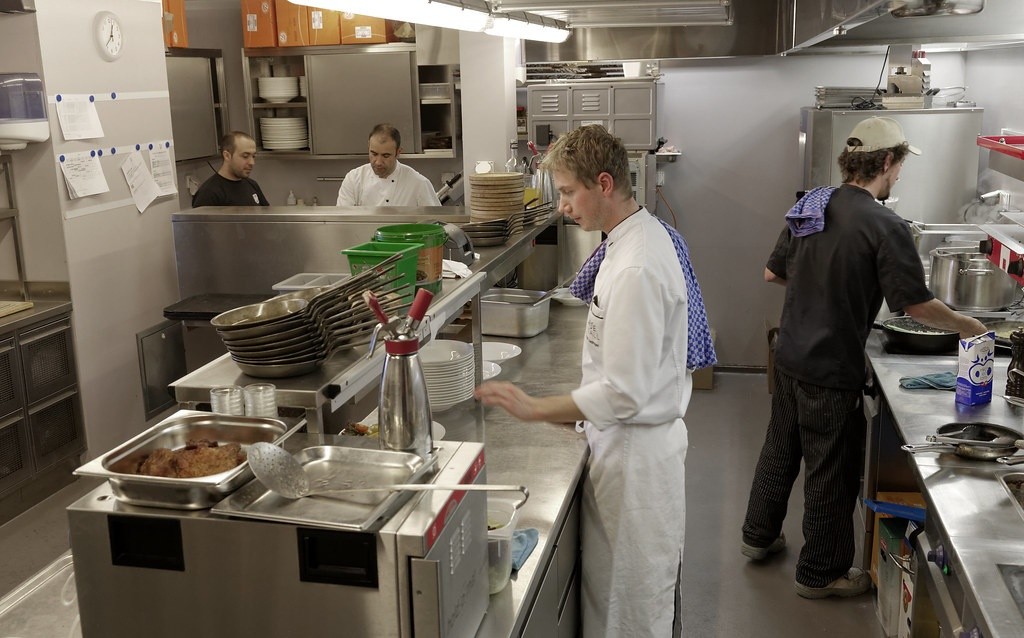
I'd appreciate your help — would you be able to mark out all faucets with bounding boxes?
[979,189,1011,212]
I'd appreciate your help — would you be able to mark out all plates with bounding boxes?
[260,117,308,150]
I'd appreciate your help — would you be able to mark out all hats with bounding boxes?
[846,115,923,156]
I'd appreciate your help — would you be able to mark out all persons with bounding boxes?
[192,131,270,208]
[740,115,988,599]
[336,123,442,207]
[470,124,693,638]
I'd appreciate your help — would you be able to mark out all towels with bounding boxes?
[785,184,839,238]
[568,211,718,371]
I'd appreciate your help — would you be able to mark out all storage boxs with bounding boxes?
[162,0,187,48]
[870,491,926,638]
[241,0,415,48]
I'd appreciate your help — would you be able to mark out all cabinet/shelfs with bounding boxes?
[241,43,463,160]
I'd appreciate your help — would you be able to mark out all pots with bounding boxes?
[872,315,962,352]
[901,423,1024,458]
[459,197,555,246]
[211,252,413,371]
[929,246,1017,312]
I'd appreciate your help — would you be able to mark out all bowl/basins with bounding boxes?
[420,340,475,413]
[552,288,586,306]
[258,75,307,104]
[483,361,501,380]
[483,342,522,364]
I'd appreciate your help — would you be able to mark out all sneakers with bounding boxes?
[740,530,786,561]
[794,565,870,600]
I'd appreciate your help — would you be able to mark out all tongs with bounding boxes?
[927,426,1015,449]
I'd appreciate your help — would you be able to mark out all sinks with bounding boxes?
[913,223,983,234]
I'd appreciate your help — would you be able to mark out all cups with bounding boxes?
[210,383,279,420]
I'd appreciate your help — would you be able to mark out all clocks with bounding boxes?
[93,11,125,62]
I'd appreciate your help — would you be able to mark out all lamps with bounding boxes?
[287,0,574,43]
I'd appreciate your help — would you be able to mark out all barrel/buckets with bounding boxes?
[370,223,449,293]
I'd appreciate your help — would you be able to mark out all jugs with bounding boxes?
[362,288,433,456]
